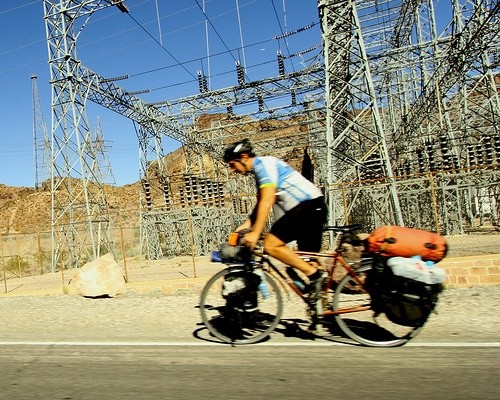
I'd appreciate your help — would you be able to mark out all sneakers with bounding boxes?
[308,270,329,301]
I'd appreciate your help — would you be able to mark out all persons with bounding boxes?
[221,138,334,311]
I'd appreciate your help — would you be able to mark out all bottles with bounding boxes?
[285,267,309,294]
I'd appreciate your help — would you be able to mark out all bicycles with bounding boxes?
[198,223,429,345]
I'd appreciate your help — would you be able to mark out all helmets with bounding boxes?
[224,138,254,163]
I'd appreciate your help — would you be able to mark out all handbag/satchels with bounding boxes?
[377,257,448,328]
[368,226,447,264]
